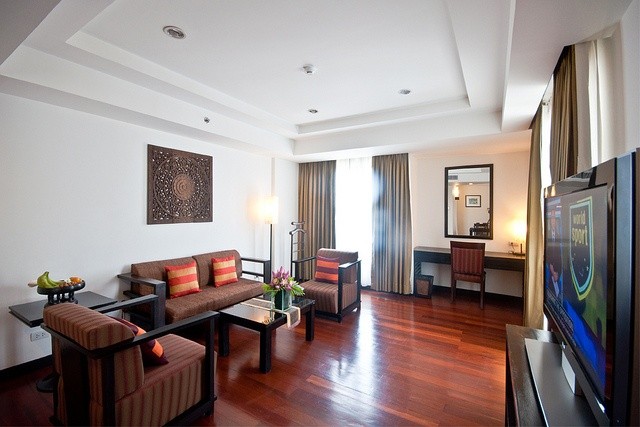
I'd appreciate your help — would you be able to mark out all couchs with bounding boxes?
[292,246,362,322]
[117,247,273,330]
[41,293,218,426]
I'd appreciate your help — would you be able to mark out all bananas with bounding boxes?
[37,271,64,288]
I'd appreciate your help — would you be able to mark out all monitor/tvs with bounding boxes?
[523,147,640,427]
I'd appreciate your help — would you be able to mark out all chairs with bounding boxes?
[449,239,486,309]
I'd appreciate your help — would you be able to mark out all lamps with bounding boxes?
[253,195,280,280]
[452,186,460,200]
[510,218,526,256]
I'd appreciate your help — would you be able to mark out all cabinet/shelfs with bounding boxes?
[504,321,561,426]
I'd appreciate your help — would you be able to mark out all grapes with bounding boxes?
[59,281,77,288]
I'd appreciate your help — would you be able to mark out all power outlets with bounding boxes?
[31,329,50,341]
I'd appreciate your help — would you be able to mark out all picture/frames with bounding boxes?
[465,194,481,207]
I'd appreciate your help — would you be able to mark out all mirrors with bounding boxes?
[444,163,494,239]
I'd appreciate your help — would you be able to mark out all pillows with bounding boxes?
[314,253,340,285]
[108,314,169,365]
[164,259,203,299]
[211,255,239,288]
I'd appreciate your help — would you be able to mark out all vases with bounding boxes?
[274,288,290,310]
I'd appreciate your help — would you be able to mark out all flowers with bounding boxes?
[262,265,306,296]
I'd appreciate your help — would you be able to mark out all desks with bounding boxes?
[6,290,118,393]
[413,245,524,308]
